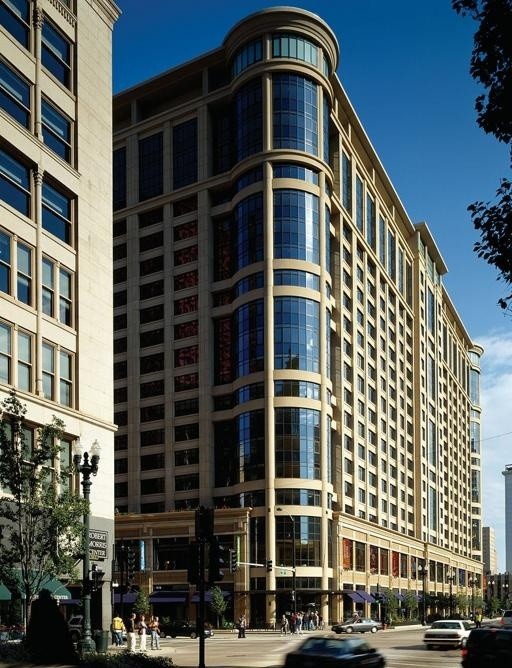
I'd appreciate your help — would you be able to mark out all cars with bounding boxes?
[332,615,383,634]
[158,619,214,639]
[421,610,511,667]
[285,636,386,668]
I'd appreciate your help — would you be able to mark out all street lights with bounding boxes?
[417,564,509,626]
[73,432,101,657]
[276,505,297,615]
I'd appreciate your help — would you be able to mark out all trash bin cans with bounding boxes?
[93,630,109,653]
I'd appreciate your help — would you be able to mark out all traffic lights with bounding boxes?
[266,558,273,574]
[127,545,137,584]
[188,505,237,586]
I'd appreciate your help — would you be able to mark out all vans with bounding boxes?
[68,614,127,643]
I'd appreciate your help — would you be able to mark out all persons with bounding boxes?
[238,614,246,639]
[110,613,161,652]
[0,622,25,641]
[475,608,483,628]
[280,608,323,636]
[353,615,360,623]
[459,612,464,620]
